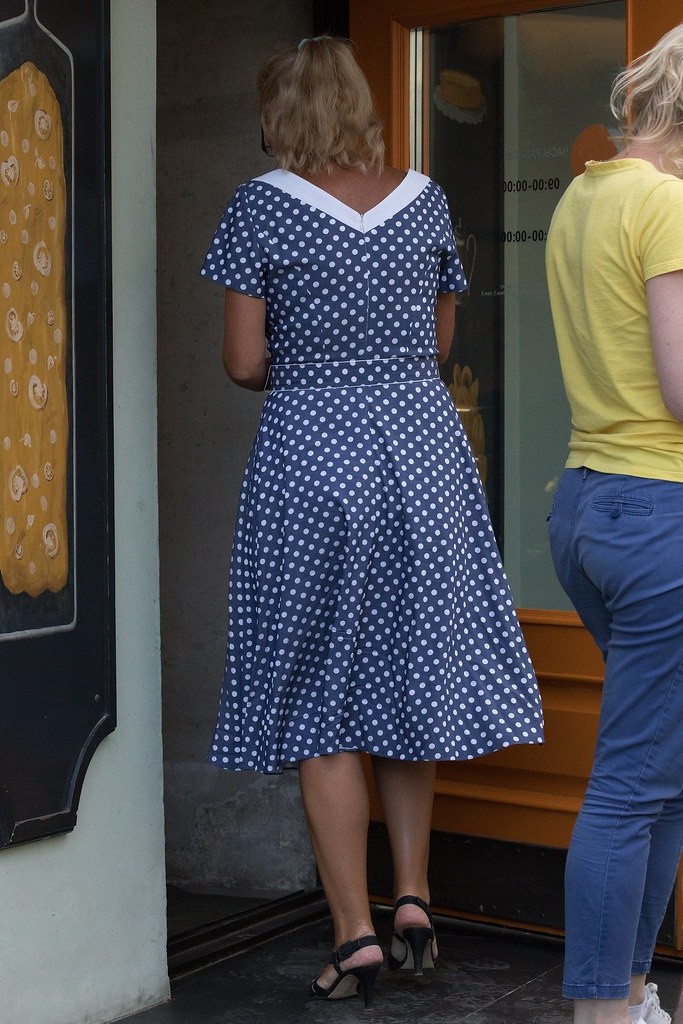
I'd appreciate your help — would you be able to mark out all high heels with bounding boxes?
[386,894,439,988]
[309,935,384,1010]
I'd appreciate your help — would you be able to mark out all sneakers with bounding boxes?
[629,982,672,1024]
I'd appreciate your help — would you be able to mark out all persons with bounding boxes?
[201,37,546,1001]
[546,25,683,1024]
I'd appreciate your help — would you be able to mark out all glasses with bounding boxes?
[261,126,274,157]
